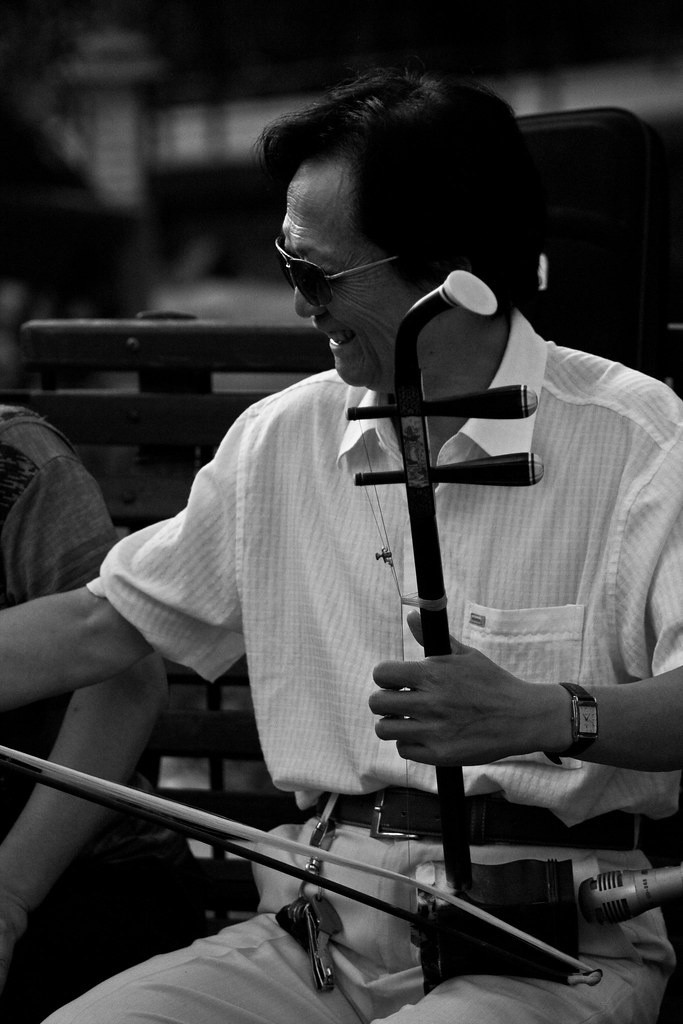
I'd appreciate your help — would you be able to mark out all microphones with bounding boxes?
[578,862,683,925]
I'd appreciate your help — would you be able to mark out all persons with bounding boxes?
[0,67,683,1024]
[0,402,205,1023]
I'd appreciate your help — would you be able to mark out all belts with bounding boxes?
[315,785,654,851]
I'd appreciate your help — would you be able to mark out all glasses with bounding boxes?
[274,234,400,307]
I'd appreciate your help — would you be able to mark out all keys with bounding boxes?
[286,893,343,955]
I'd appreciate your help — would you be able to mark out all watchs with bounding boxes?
[559,681,601,759]
